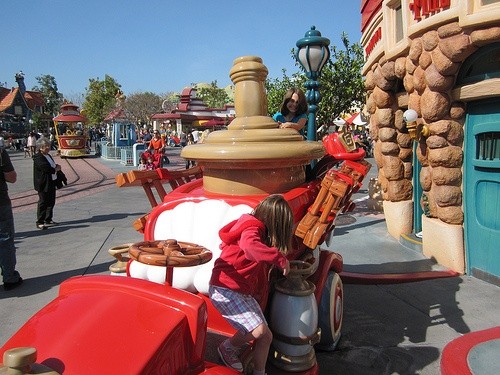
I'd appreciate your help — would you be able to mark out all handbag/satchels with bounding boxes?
[160,155,170,165]
[55,170,63,186]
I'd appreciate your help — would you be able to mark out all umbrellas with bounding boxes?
[326,110,370,133]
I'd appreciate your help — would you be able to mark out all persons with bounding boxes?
[146,157,155,170]
[147,130,165,154]
[87,126,102,141]
[272,87,308,136]
[50,132,57,150]
[135,128,152,148]
[180,130,188,148]
[138,158,147,171]
[0,147,23,290]
[208,194,293,375]
[353,128,375,158]
[0,134,5,147]
[23,132,40,159]
[32,137,61,230]
[186,133,195,167]
[191,129,199,144]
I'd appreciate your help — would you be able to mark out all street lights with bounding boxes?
[295,25,331,169]
[29,118,34,132]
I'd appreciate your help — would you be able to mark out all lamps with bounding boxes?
[403,109,429,140]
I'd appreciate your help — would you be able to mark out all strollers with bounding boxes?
[138,149,162,172]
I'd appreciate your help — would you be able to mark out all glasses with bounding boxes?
[187,136,191,138]
[154,133,158,134]
[290,99,300,105]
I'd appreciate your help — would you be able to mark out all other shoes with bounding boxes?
[4,277,22,290]
[37,224,47,230]
[218,340,243,371]
[45,221,55,224]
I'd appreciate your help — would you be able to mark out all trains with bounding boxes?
[52,101,92,159]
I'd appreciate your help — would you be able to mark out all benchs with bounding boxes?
[127,196,255,295]
